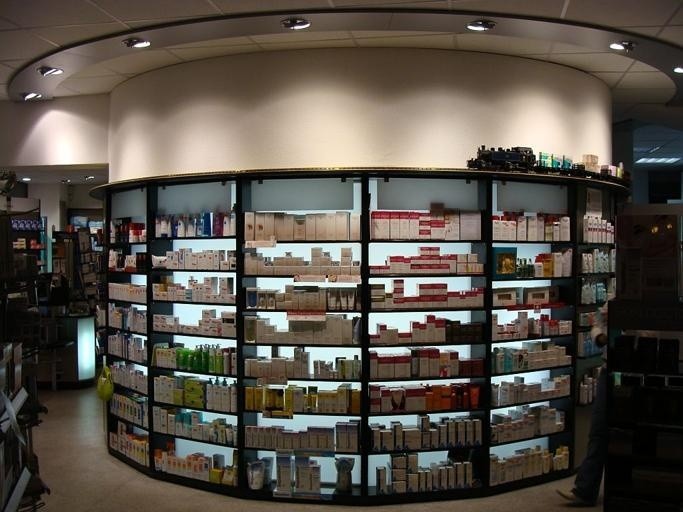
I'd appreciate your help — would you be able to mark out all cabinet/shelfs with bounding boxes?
[152,237,237,488]
[242,239,363,496]
[0,213,47,287]
[107,241,149,469]
[489,240,577,487]
[52,228,99,310]
[366,240,488,496]
[6,312,96,390]
[575,242,616,406]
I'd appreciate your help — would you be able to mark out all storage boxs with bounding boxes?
[603,386,683,512]
[616,203,683,304]
[607,300,682,375]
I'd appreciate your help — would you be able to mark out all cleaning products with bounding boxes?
[247,461,265,490]
[194,343,222,374]
[259,457,273,485]
[334,456,356,494]
[205,376,237,413]
